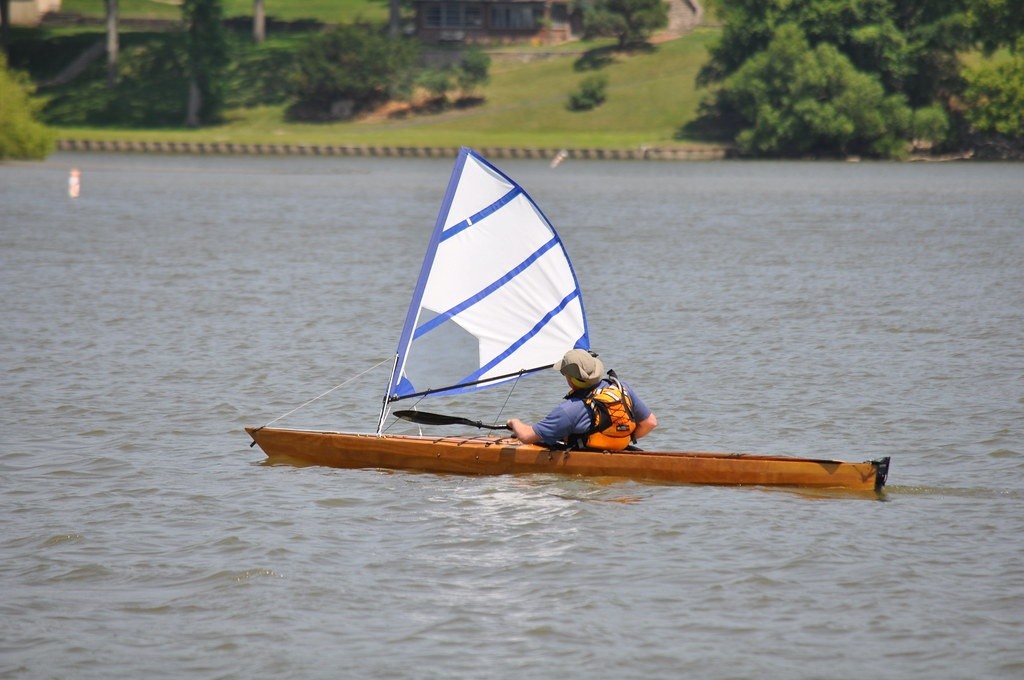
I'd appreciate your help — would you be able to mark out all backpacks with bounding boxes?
[585,382,636,452]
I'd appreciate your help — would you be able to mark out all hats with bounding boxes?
[553,349,604,385]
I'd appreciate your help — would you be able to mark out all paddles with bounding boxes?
[392,409,513,433]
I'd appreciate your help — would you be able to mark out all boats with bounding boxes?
[242,145,891,494]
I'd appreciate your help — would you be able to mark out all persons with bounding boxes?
[506,349,657,452]
